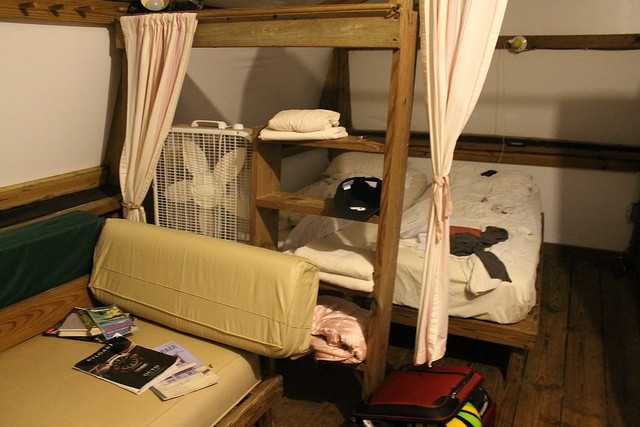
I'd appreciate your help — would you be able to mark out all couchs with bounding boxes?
[0,209,287,427]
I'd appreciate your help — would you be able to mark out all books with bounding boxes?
[145,341,218,400]
[43,304,137,344]
[73,333,180,394]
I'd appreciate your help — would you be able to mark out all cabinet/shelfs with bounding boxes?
[338,0,640,262]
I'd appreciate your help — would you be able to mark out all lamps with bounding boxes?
[139,0,171,13]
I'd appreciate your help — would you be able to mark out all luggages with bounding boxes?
[350,364,496,427]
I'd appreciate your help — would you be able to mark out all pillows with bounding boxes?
[90,219,318,361]
[322,151,406,182]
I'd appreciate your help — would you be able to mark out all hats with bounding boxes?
[333,177,382,221]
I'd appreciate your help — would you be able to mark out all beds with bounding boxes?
[291,148,543,391]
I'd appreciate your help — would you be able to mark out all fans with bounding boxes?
[147,123,253,243]
